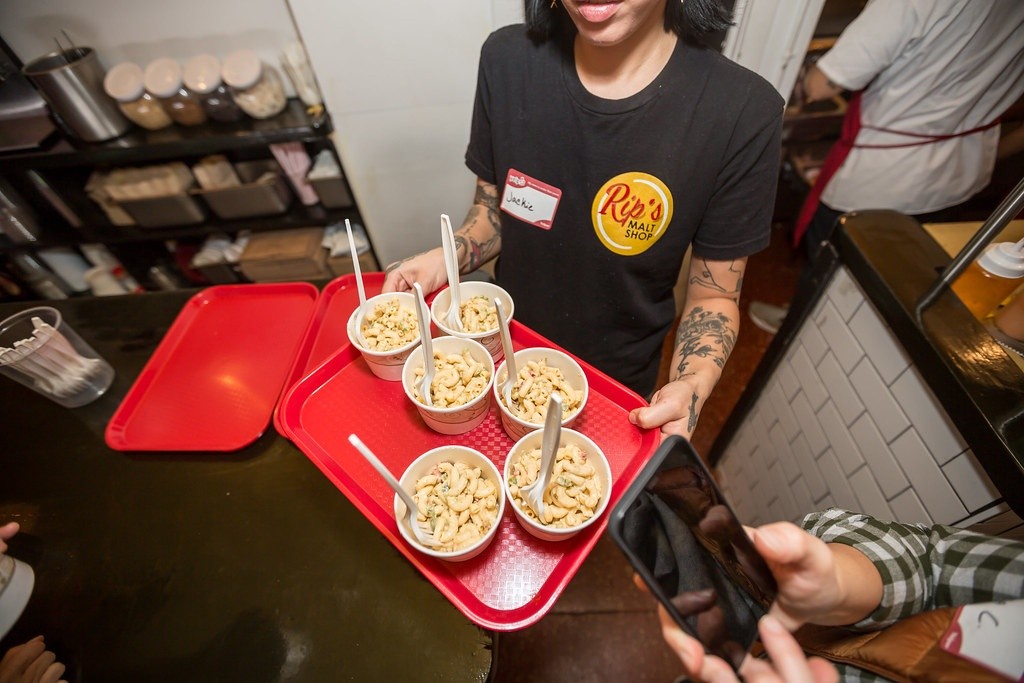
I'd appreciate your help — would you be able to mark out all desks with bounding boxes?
[0,279,495,683]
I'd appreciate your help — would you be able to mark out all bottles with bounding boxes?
[182,52,244,124]
[144,57,204,126]
[950,236,1024,320]
[104,62,172,130]
[220,49,288,120]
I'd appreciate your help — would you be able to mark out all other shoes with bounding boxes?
[749,301,790,336]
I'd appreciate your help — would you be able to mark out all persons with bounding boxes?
[635,507,1024,683]
[0,521,67,683]
[749,0,1024,334]
[619,441,774,670]
[382,1,786,442]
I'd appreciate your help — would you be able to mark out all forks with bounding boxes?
[348,433,442,548]
[440,213,465,331]
[414,282,435,407]
[0,319,102,397]
[492,297,520,417]
[344,218,367,350]
[520,393,563,525]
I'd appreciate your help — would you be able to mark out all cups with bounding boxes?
[0,306,114,408]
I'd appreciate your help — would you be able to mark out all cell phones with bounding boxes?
[606,434,779,674]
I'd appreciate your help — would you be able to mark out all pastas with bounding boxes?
[508,443,600,530]
[500,356,581,425]
[361,304,419,352]
[440,295,499,333]
[413,351,490,409]
[410,461,498,551]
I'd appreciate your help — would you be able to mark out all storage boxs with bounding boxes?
[107,185,204,226]
[242,222,330,281]
[193,156,291,218]
[326,248,376,278]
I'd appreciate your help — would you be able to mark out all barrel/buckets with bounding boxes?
[21,46,128,143]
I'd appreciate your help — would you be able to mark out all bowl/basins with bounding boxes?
[494,347,589,441]
[504,426,614,541]
[430,281,515,364]
[392,445,505,564]
[346,292,430,382]
[402,335,494,435]
